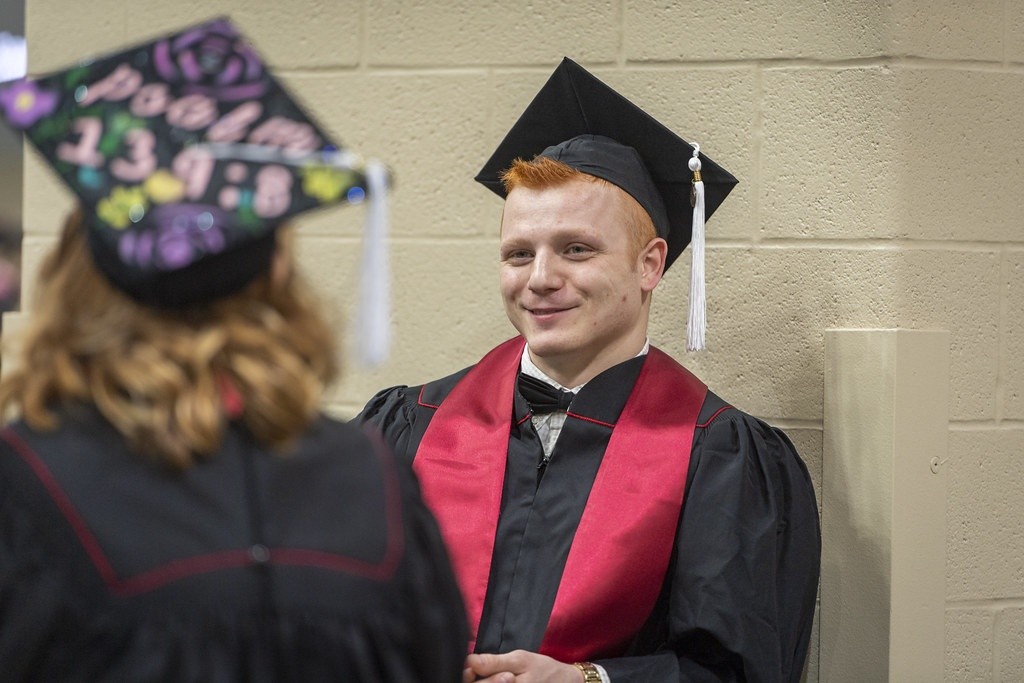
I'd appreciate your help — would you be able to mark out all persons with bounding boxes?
[0,11,473,683]
[341,127,822,683]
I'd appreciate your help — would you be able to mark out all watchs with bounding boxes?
[574,659,604,683]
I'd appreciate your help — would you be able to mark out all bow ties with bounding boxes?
[519,373,574,413]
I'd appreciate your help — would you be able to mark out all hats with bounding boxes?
[1,12,392,384]
[474,57,738,350]
